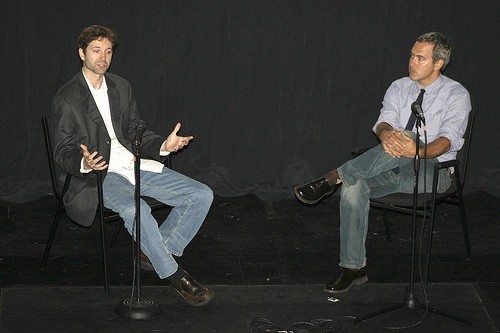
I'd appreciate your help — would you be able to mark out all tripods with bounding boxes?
[352,118,473,327]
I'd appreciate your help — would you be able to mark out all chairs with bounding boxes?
[38,114,173,295]
[351,106,476,299]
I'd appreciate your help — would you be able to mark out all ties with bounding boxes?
[405,88,426,131]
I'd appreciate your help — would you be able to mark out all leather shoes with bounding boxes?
[139,250,154,271]
[323,265,368,293]
[294,174,338,205]
[168,270,214,307]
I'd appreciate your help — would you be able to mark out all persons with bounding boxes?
[293,33,472,293]
[50,25,215,305]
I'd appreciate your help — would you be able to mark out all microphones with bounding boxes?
[134,120,147,158]
[411,102,426,125]
